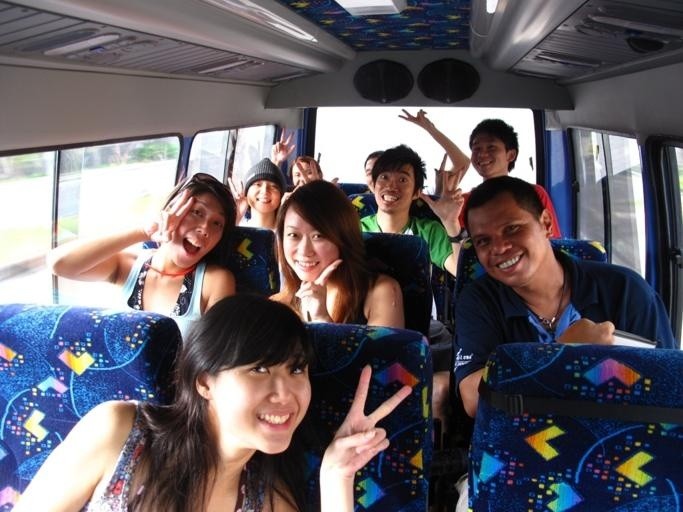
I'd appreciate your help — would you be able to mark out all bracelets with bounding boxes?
[444,226,464,243]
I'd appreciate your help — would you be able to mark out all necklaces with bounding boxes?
[523,267,570,331]
[143,260,198,277]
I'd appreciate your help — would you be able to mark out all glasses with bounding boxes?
[173,172,233,205]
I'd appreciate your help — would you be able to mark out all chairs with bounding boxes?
[455,235,615,300]
[143,225,282,296]
[1,299,184,512]
[468,334,682,512]
[277,320,431,512]
[336,182,453,327]
[357,229,433,351]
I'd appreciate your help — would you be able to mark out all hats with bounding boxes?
[244,158,287,197]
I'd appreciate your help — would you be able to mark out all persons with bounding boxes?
[223,155,300,231]
[294,142,467,452]
[363,107,472,190]
[450,174,680,421]
[268,127,325,188]
[433,118,563,240]
[8,293,415,512]
[251,178,406,331]
[41,172,238,353]
